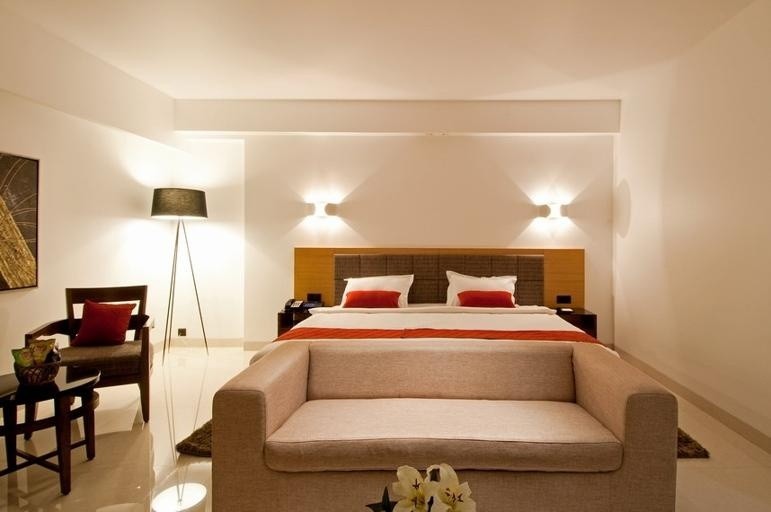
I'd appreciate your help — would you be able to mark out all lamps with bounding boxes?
[149,371,211,512]
[535,203,570,220]
[149,186,210,365]
[301,200,341,220]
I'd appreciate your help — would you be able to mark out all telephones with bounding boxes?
[285,299,302,309]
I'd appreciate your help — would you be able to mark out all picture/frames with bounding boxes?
[0,151,40,293]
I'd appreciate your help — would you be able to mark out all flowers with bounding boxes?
[364,460,480,512]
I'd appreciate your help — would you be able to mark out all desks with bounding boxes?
[0,366,103,495]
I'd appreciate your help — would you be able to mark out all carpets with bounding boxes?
[173,418,212,458]
[676,427,714,462]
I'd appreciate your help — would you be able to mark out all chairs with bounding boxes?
[24,284,151,443]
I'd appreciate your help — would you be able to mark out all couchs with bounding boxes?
[210,337,679,512]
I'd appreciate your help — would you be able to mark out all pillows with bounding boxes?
[340,273,417,308]
[458,290,517,310]
[343,291,403,311]
[70,300,138,348]
[444,269,518,307]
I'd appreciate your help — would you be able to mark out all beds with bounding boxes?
[249,253,624,363]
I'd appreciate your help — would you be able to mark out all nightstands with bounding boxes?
[554,305,598,339]
[277,304,323,339]
